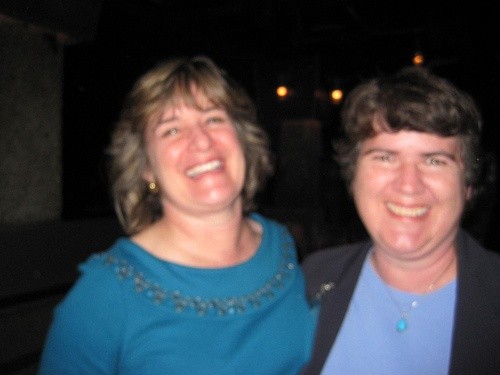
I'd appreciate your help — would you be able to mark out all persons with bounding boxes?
[293,66,498,375]
[35,54,323,375]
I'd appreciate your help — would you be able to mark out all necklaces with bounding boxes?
[373,249,457,333]
